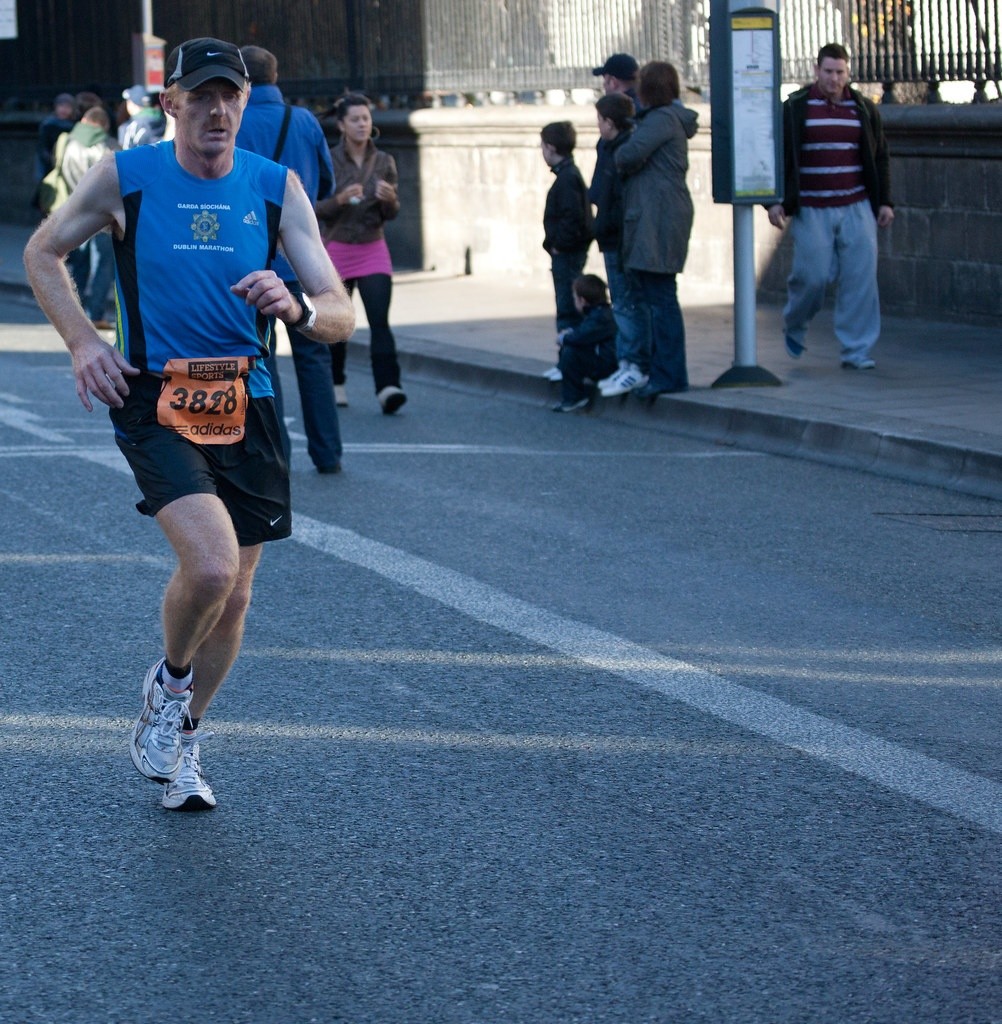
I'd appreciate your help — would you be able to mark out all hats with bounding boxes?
[121,85,152,106]
[593,54,637,79]
[164,37,249,91]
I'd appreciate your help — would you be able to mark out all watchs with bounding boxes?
[291,291,312,328]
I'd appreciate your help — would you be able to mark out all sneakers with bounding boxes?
[597,361,628,388]
[600,363,650,397]
[162,731,215,810]
[130,656,194,782]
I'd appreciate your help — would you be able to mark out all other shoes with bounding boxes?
[557,396,589,411]
[842,357,875,370]
[542,366,564,380]
[333,384,348,406]
[378,386,406,414]
[785,337,802,358]
[92,320,114,329]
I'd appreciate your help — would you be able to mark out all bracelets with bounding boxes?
[297,306,316,333]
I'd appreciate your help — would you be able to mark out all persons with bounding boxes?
[606,62,700,402]
[763,43,896,370]
[31,90,101,218]
[23,38,357,809]
[117,84,166,149]
[52,105,122,331]
[315,93,407,415]
[541,121,592,381]
[593,54,643,116]
[592,92,648,397]
[551,273,618,412]
[233,46,344,475]
